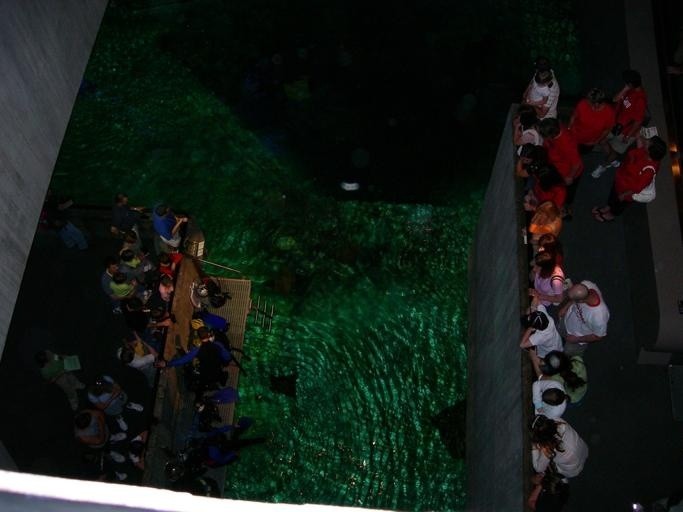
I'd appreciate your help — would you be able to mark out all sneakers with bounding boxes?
[143,264,152,272]
[126,402,144,412]
[143,290,153,312]
[115,414,128,431]
[109,433,127,441]
[113,306,122,314]
[592,160,620,178]
[109,450,128,481]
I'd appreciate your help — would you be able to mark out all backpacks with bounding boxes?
[162,313,176,324]
[207,279,225,308]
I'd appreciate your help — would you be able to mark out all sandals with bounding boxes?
[591,204,615,222]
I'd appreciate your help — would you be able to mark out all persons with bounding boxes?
[12,187,264,491]
[511,31,672,512]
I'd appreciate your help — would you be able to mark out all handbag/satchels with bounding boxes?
[611,123,622,136]
[632,166,657,204]
[159,235,181,248]
[550,276,573,306]
